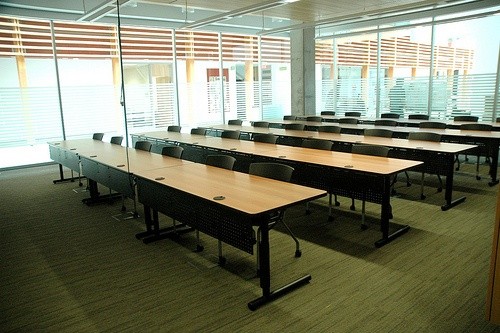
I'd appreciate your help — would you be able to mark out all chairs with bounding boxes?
[94,136,123,194]
[168,153,236,252]
[321,110,479,123]
[143,145,184,227]
[318,125,341,133]
[167,126,181,131]
[285,122,306,130]
[363,129,394,138]
[252,122,270,128]
[328,144,392,231]
[339,118,358,124]
[301,138,334,151]
[420,121,445,129]
[252,132,276,143]
[70,132,104,188]
[374,120,396,126]
[221,130,242,139]
[120,141,153,214]
[306,116,322,121]
[393,131,446,200]
[227,119,243,125]
[219,160,304,266]
[283,115,297,120]
[191,128,206,135]
[456,122,496,181]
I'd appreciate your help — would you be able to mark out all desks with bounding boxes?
[47,115,500,313]
